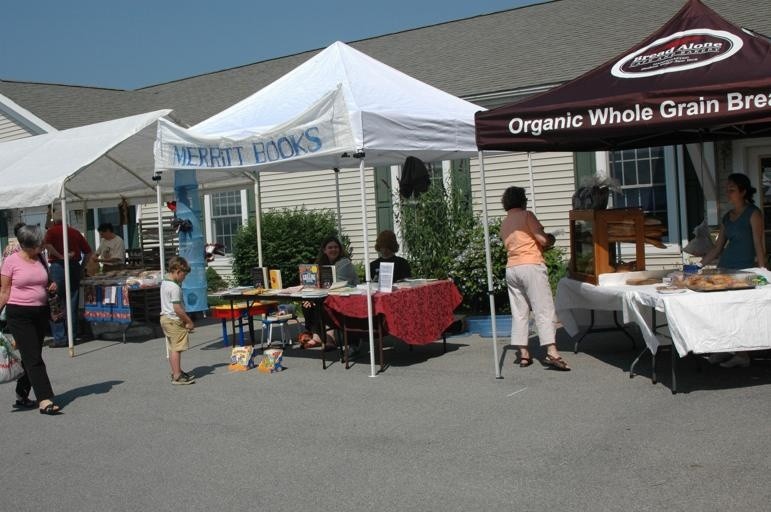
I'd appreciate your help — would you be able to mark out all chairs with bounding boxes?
[256,302,303,350]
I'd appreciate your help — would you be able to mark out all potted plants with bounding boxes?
[444,214,570,340]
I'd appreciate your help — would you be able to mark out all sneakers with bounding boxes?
[172,371,196,379]
[720,355,752,369]
[171,375,195,384]
[50,342,66,347]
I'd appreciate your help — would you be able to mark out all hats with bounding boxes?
[375,230,399,252]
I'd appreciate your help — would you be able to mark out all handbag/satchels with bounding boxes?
[39,254,56,298]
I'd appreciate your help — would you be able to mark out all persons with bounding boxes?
[43,209,93,348]
[1,223,26,262]
[92,223,125,272]
[500,186,570,370]
[697,173,766,368]
[301,237,360,349]
[0,225,61,413]
[160,256,196,385]
[365,230,411,281]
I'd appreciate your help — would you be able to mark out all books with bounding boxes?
[213,263,438,298]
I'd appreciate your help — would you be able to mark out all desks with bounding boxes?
[549,262,726,394]
[633,266,771,395]
[209,297,284,349]
[316,276,467,373]
[78,267,168,345]
[202,274,356,374]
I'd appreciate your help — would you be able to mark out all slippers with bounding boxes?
[13,396,38,408]
[40,402,61,414]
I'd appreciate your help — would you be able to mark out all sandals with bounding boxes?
[520,358,534,367]
[544,353,571,371]
[304,338,322,348]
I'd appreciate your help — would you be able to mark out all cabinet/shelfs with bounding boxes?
[565,203,648,290]
